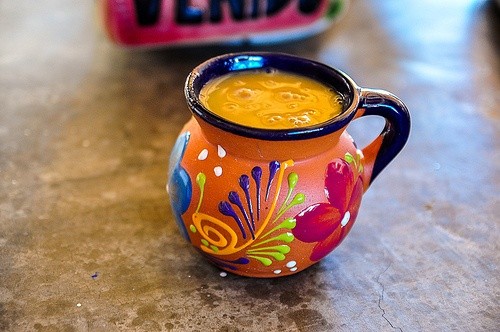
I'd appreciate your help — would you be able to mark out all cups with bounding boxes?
[164,51,413,280]
